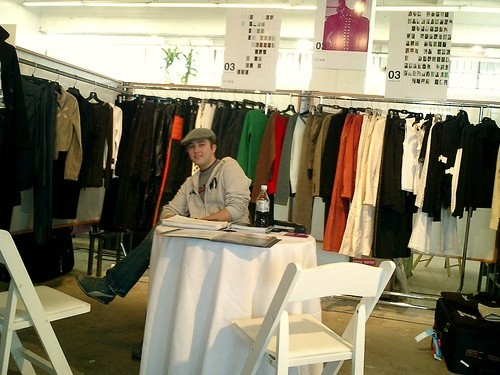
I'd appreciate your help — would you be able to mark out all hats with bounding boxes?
[181,128,216,148]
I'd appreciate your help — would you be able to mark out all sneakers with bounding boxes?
[75,272,115,304]
[131,340,144,360]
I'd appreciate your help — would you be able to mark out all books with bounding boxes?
[159,229,281,248]
[160,214,229,231]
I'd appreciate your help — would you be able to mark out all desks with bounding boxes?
[139,231,325,375]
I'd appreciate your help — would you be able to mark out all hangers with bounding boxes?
[32,62,112,104]
[117,84,500,124]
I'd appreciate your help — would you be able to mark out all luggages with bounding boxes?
[433,291,500,375]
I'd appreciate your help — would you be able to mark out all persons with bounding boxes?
[76,128,252,359]
[323,0,369,53]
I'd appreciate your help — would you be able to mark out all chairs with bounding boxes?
[0,229,91,375]
[230,261,396,375]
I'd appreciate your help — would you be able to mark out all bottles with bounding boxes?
[255,185,270,227]
